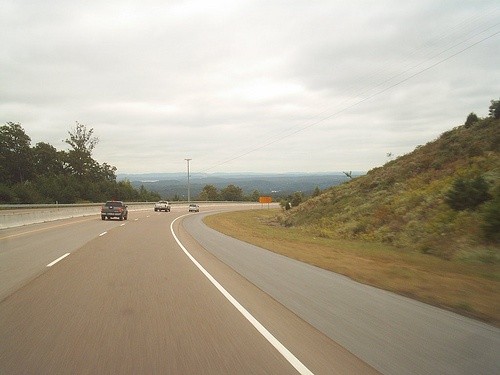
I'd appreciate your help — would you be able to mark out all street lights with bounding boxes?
[185,159,192,202]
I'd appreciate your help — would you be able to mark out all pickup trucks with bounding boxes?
[101,201,128,221]
[154,200,170,211]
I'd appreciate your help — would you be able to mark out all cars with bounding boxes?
[189,204,199,213]
